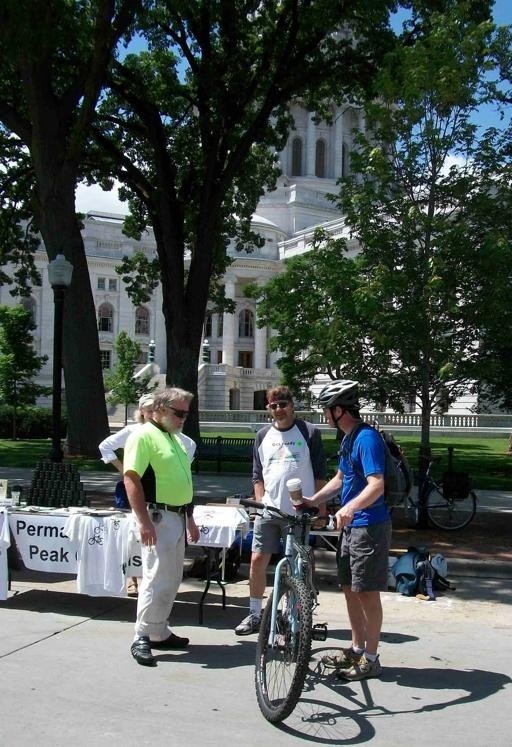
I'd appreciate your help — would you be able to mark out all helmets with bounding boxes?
[313,379,358,408]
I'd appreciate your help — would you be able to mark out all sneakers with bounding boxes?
[130,636,154,664]
[150,633,189,648]
[324,647,382,681]
[235,609,265,635]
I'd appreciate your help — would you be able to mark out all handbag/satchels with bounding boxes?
[391,551,448,596]
[190,556,239,580]
[115,483,130,507]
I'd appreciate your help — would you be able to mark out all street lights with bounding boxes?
[40,252,78,463]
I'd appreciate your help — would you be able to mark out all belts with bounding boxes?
[145,502,188,513]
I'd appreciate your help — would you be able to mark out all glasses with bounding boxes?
[270,401,290,409]
[163,403,189,419]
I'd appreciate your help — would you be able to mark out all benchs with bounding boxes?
[191,438,253,478]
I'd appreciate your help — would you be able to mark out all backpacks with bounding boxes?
[347,419,413,507]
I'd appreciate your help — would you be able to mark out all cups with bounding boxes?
[285,477,304,505]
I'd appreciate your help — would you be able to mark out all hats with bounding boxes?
[139,393,158,408]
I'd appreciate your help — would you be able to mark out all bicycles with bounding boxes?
[385,453,481,533]
[233,500,349,727]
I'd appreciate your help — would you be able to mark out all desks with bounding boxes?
[0,503,247,626]
[217,521,343,584]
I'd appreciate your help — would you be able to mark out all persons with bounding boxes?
[290,380,392,682]
[234,385,329,637]
[122,388,200,664]
[97,395,198,597]
